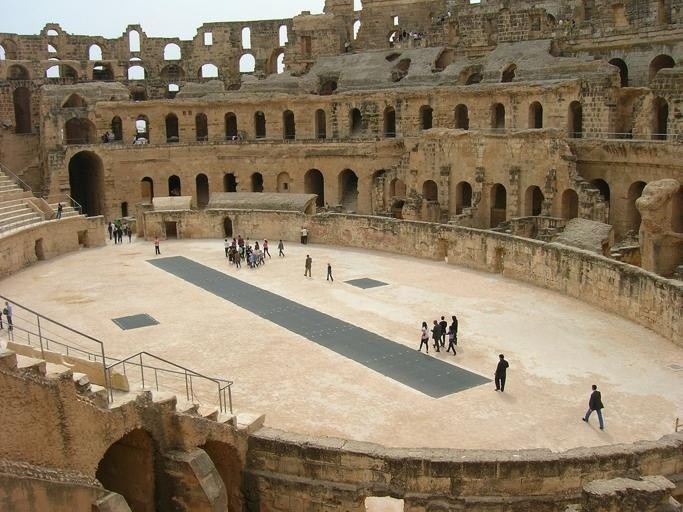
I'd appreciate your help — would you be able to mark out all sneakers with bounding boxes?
[600,426,604,429]
[226,255,270,268]
[156,252,160,255]
[110,238,131,244]
[583,418,588,423]
[417,350,428,354]
[446,350,456,355]
[433,344,440,352]
[494,388,504,392]
[279,254,284,257]
[441,343,444,347]
[304,274,311,277]
[327,278,333,282]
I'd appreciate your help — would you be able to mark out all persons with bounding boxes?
[4,301,13,331]
[438,316,447,346]
[154,236,160,255]
[303,254,312,277]
[300,228,308,245]
[582,384,604,429]
[55,203,62,219]
[107,219,132,244]
[222,234,272,268]
[417,322,429,353]
[277,239,285,256]
[451,315,458,345]
[429,320,442,352]
[494,354,509,392]
[442,326,457,355]
[101,128,115,143]
[0,310,3,329]
[326,263,333,282]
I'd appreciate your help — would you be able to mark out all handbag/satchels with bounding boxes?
[453,331,457,345]
